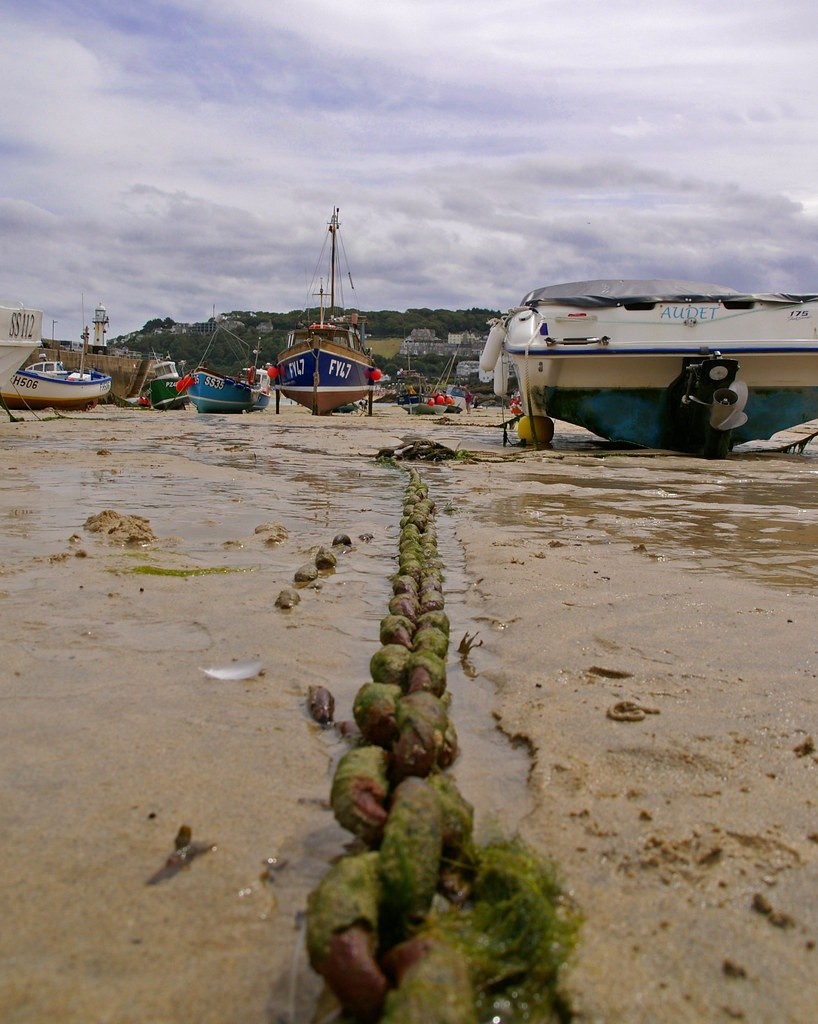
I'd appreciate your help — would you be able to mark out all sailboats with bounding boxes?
[274,205,377,415]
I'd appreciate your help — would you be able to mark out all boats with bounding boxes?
[141,361,189,410]
[396,403,447,415]
[502,280,818,460]
[440,386,466,413]
[183,337,271,413]
[0,299,43,391]
[396,343,435,405]
[0,326,112,411]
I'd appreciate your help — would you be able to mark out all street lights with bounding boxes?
[52,320,58,349]
